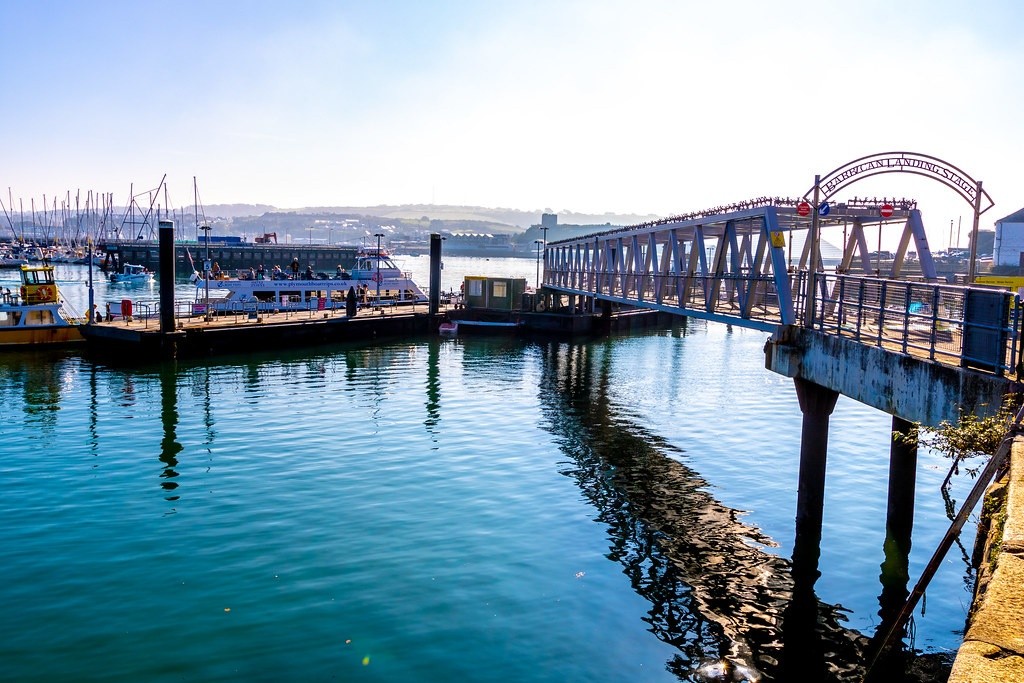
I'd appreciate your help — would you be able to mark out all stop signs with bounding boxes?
[881,204,893,218]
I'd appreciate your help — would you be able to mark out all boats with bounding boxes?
[0,172,430,348]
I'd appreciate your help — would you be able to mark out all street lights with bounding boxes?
[534,240,544,288]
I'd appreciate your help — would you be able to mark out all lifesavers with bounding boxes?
[372,271,383,282]
[37,285,52,300]
[20,286,26,300]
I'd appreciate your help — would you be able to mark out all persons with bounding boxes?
[105,256,368,321]
[461,281,465,300]
[57,238,76,248]
[0,287,13,302]
[85,304,102,323]
[2,252,24,260]
[524,281,531,290]
[559,297,564,307]
